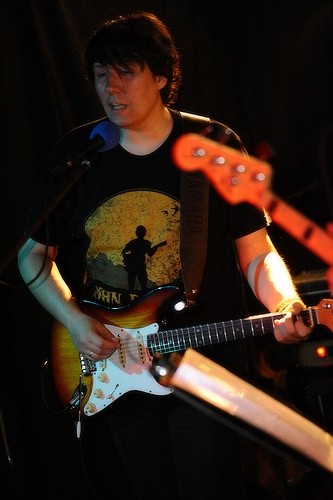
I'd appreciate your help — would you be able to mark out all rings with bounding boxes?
[89,351,94,357]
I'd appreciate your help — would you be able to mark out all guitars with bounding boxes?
[49,285,333,419]
[173,126,333,295]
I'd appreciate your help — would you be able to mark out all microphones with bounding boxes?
[59,121,122,173]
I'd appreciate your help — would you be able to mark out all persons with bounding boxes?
[18,11,315,363]
